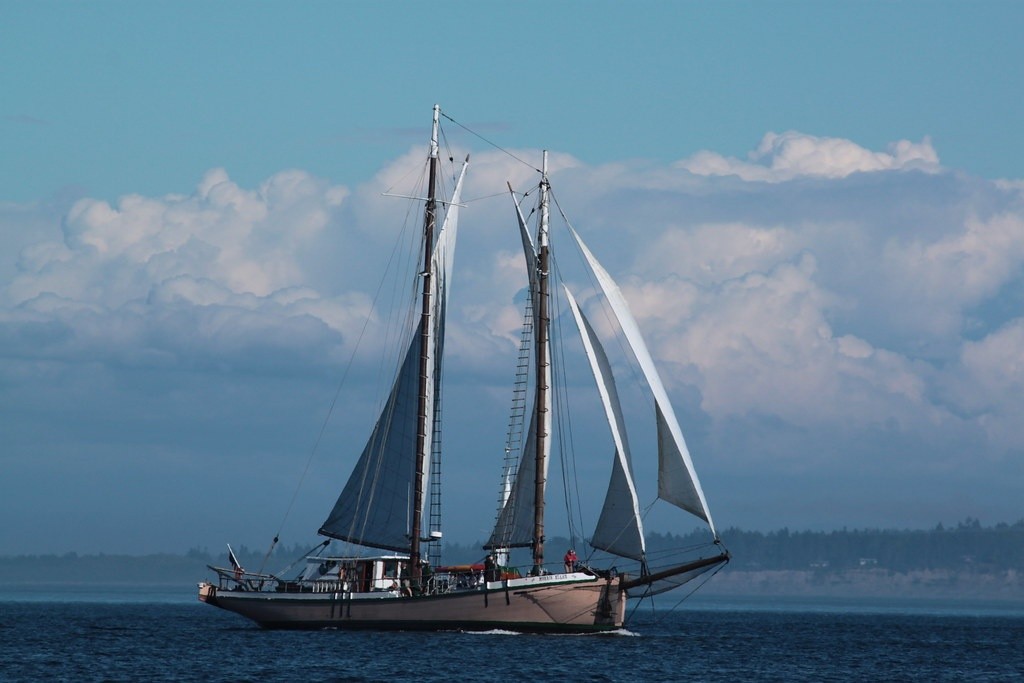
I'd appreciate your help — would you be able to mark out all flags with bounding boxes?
[229,552,242,578]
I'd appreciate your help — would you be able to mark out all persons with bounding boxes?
[483,555,496,583]
[564,549,579,573]
[421,561,434,594]
[400,563,412,597]
[452,573,478,589]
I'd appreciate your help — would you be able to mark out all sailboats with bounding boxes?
[197,103,733,633]
